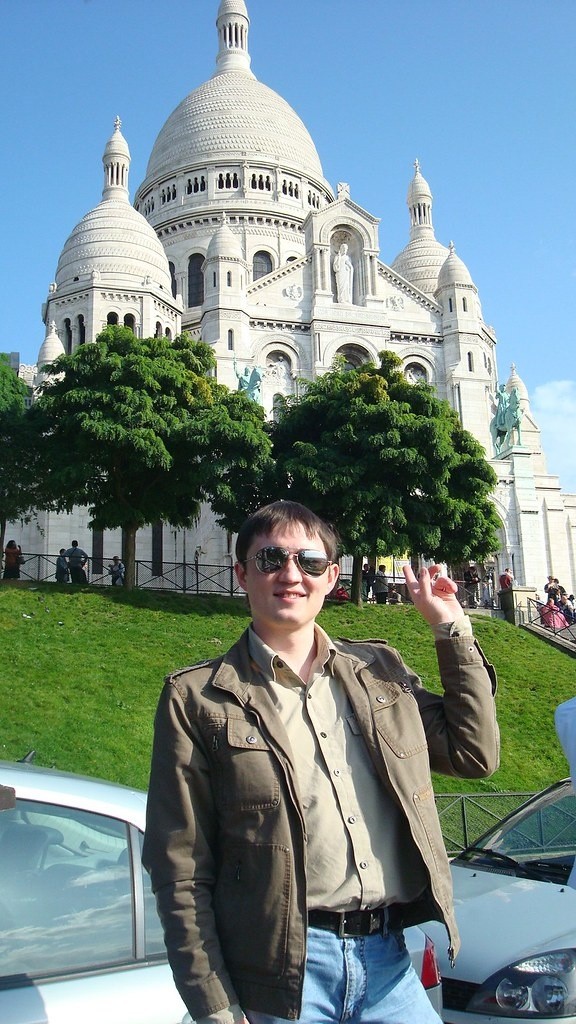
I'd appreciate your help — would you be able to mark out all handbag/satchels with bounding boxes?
[116,577,124,586]
[17,553,25,565]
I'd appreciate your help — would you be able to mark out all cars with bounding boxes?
[0,751,444,1024]
[413,777,576,1024]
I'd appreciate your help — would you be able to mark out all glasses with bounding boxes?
[242,545,333,577]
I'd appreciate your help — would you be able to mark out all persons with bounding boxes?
[495,385,510,427]
[56,540,88,583]
[464,567,490,608]
[374,565,388,605]
[4,540,21,579]
[109,556,125,585]
[335,585,349,600]
[142,501,500,1024]
[333,244,353,304]
[536,576,576,627]
[499,568,512,589]
[388,586,401,605]
[233,361,258,402]
[362,564,371,603]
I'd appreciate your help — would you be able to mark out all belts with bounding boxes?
[308,901,404,938]
